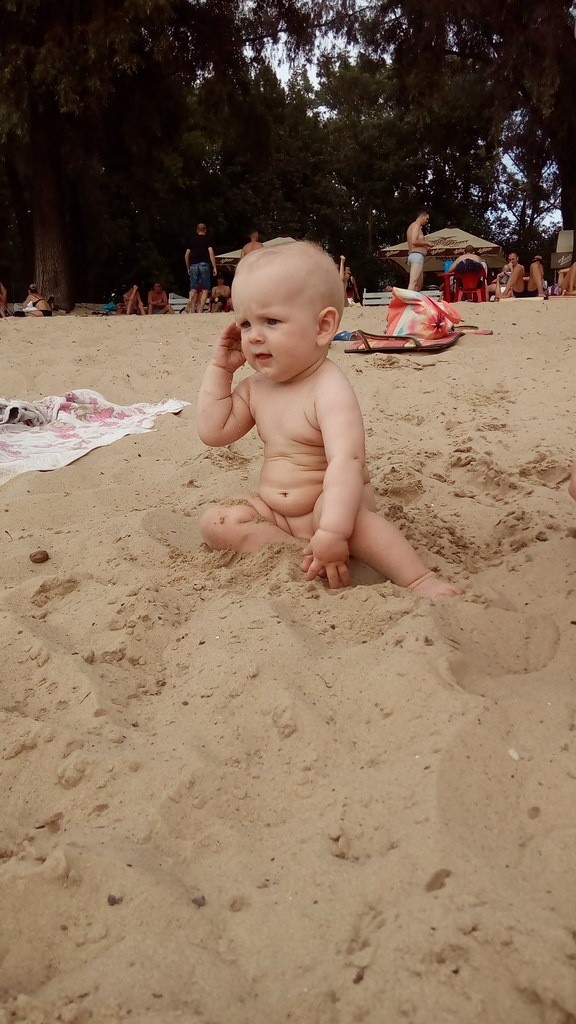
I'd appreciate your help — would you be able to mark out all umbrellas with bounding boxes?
[215,236,297,266]
[376,225,510,274]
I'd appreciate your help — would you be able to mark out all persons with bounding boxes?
[148,282,175,314]
[486,253,524,300]
[209,272,230,313]
[449,245,487,300]
[185,224,218,313]
[123,283,145,315]
[333,255,360,307]
[23,284,52,316]
[241,231,263,258]
[496,262,546,298]
[545,263,576,296]
[0,281,7,317]
[197,236,465,595]
[407,211,434,291]
[533,256,544,285]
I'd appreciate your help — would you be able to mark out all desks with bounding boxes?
[437,272,457,302]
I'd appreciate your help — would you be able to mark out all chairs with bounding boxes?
[453,257,486,302]
